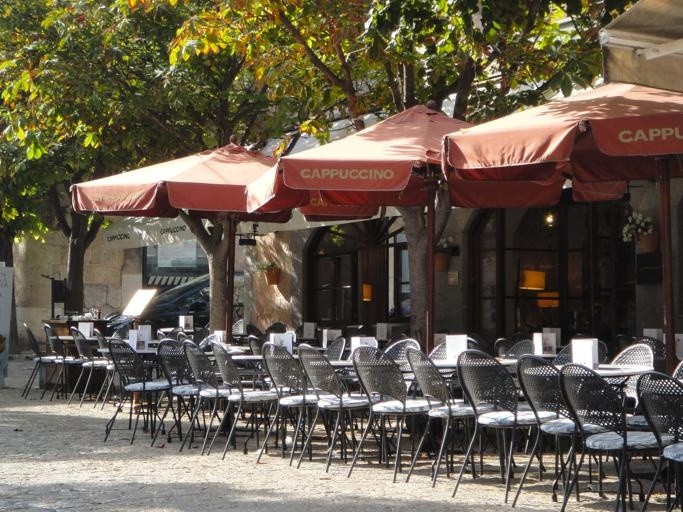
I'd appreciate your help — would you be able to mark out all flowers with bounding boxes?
[256,261,275,272]
[622,209,654,241]
[435,236,453,252]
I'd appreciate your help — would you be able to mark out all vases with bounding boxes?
[264,272,280,285]
[637,233,659,253]
[434,253,449,272]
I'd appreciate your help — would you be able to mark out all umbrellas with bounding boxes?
[277,100,627,357]
[70,133,440,342]
[442,81,683,376]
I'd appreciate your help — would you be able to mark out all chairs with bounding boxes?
[257,322,683,512]
[21,320,276,466]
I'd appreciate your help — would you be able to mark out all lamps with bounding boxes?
[519,270,546,291]
[537,292,559,308]
[363,283,372,301]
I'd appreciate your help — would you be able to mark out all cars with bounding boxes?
[101,272,210,339]
